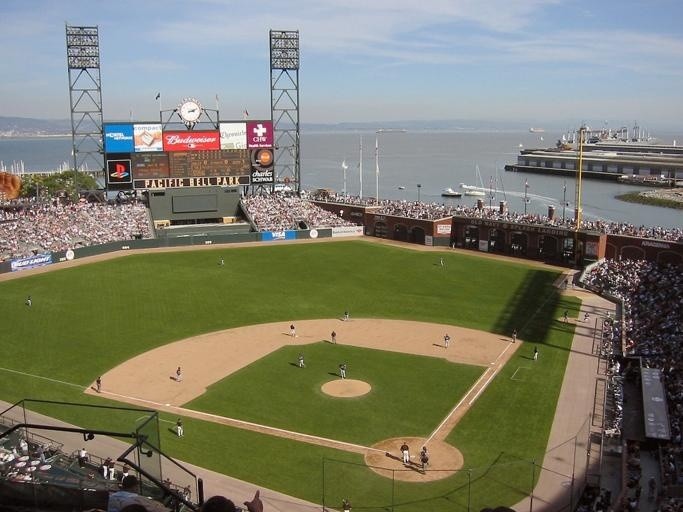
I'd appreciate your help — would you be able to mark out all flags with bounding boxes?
[244,107,249,118]
[155,92,159,100]
[215,94,219,103]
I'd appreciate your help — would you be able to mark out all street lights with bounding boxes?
[562,184,567,220]
[417,183,423,201]
[64,20,109,195]
[488,175,494,211]
[268,28,301,196]
[522,176,529,215]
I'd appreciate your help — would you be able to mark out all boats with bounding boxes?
[397,186,405,191]
[375,127,409,134]
[529,127,544,133]
[459,182,467,189]
[440,187,463,198]
[575,123,627,133]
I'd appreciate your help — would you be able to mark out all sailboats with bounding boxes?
[464,162,487,197]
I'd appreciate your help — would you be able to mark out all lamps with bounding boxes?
[82,432,94,441]
[139,445,153,458]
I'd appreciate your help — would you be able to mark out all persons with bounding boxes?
[219,256,224,267]
[0,190,157,260]
[533,345,538,360]
[377,199,682,242]
[595,437,682,510]
[343,311,349,320]
[95,376,101,392]
[290,324,295,337]
[440,257,443,268]
[176,418,183,438]
[444,332,449,348]
[400,441,429,474]
[240,192,379,233]
[339,363,347,378]
[342,499,352,510]
[330,331,336,343]
[511,329,517,342]
[582,258,682,435]
[0,436,264,511]
[25,295,31,307]
[297,352,304,367]
[177,366,181,380]
[564,310,569,325]
